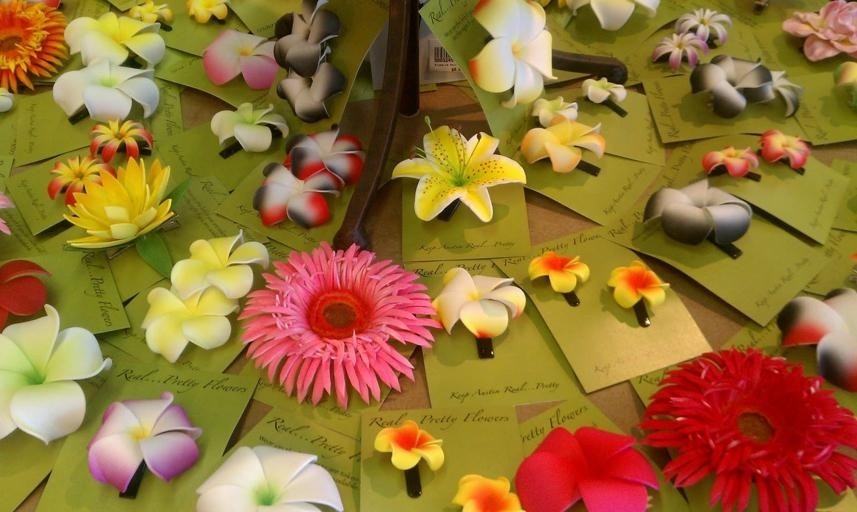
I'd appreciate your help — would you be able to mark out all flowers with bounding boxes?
[0,1,857,512]
